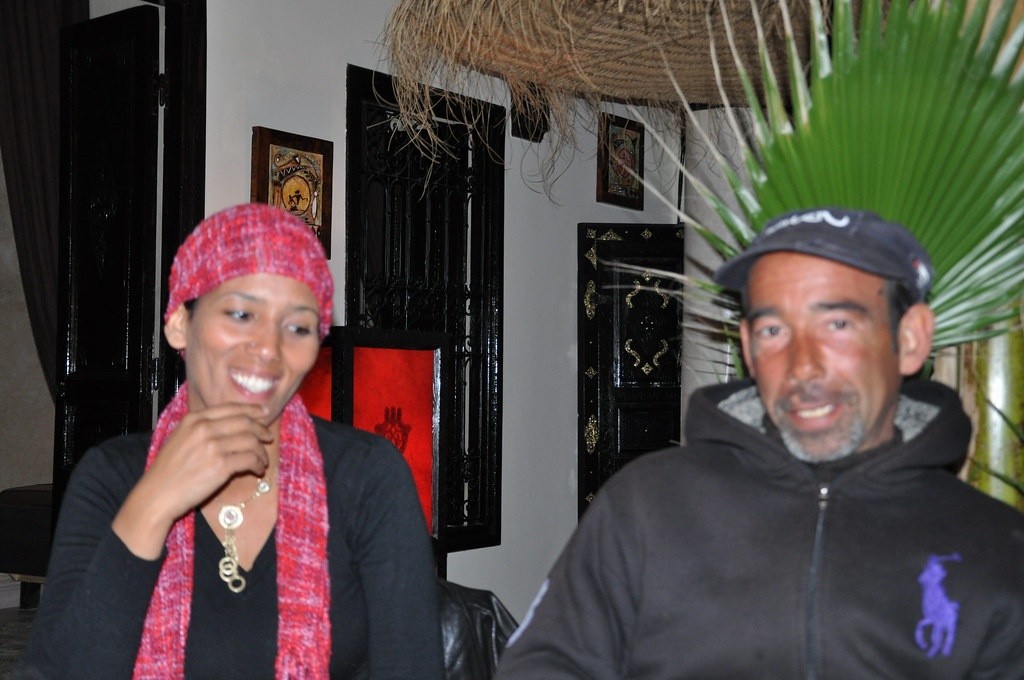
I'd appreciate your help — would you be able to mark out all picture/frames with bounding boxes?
[250,125,334,262]
[595,111,645,212]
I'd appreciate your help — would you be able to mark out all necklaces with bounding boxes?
[211,464,279,594]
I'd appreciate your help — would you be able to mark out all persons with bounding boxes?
[494,208,1024,680]
[14,204,446,680]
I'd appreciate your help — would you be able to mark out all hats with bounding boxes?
[712,210,933,298]
